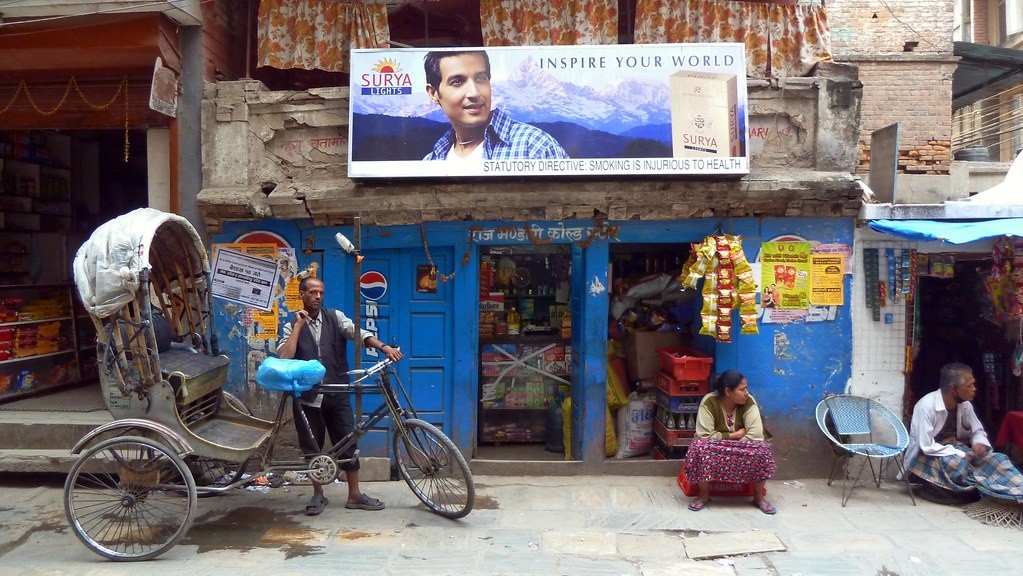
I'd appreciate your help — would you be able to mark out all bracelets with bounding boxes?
[380,344,388,352]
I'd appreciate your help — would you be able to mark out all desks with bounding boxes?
[994,411,1023,460]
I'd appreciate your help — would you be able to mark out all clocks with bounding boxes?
[511,268,532,288]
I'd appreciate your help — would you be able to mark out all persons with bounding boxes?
[684,369,776,514]
[277,277,402,515]
[897,363,1023,504]
[423,51,569,160]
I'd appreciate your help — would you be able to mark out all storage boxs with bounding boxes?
[623,330,766,495]
[479,257,571,441]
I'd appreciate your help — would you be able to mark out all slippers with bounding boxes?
[687,497,712,512]
[304,493,328,516]
[344,493,385,510]
[751,499,777,515]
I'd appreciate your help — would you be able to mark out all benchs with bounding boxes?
[104,317,229,405]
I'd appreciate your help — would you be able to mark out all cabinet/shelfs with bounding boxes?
[481,339,573,447]
[0,128,147,403]
[480,259,557,297]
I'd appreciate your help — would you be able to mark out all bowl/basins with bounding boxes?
[419,273,436,292]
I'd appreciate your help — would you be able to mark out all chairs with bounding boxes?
[815,394,916,508]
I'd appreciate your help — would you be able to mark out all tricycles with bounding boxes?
[61,208,478,559]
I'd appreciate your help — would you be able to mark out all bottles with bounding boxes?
[656,405,696,430]
[429,267,436,293]
[334,233,365,263]
[544,392,566,452]
[507,307,520,335]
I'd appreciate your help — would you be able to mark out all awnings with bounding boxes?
[868,217,1023,244]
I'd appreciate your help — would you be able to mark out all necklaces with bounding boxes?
[456,132,481,144]
